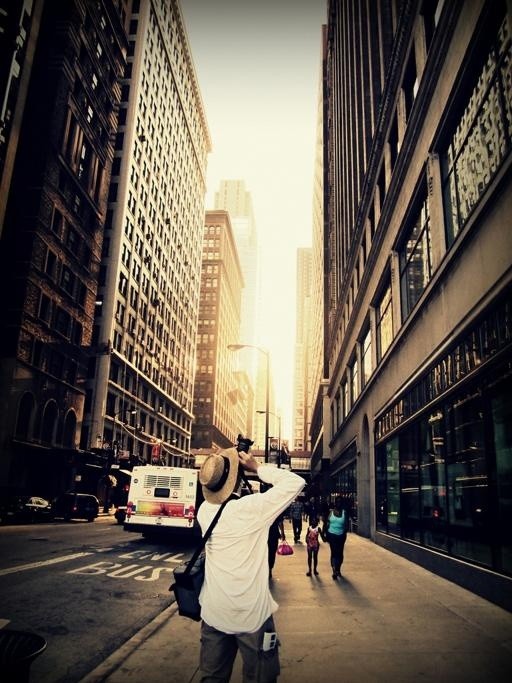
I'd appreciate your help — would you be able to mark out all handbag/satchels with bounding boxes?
[277,539,293,555]
[168,555,206,622]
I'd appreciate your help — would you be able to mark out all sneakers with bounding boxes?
[332,571,341,580]
[307,571,318,576]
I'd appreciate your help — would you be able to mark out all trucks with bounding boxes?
[124,465,201,528]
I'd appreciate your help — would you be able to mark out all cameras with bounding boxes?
[236,434,254,468]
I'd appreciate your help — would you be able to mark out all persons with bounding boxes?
[306,516,326,578]
[321,496,349,580]
[287,496,334,544]
[241,483,254,497]
[259,484,285,580]
[197,447,305,682]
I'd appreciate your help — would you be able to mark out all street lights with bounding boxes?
[160,438,178,465]
[111,409,137,450]
[228,344,281,467]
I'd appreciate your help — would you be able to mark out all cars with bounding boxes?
[115,507,127,522]
[26,492,99,522]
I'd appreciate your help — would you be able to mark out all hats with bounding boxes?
[198,447,240,505]
